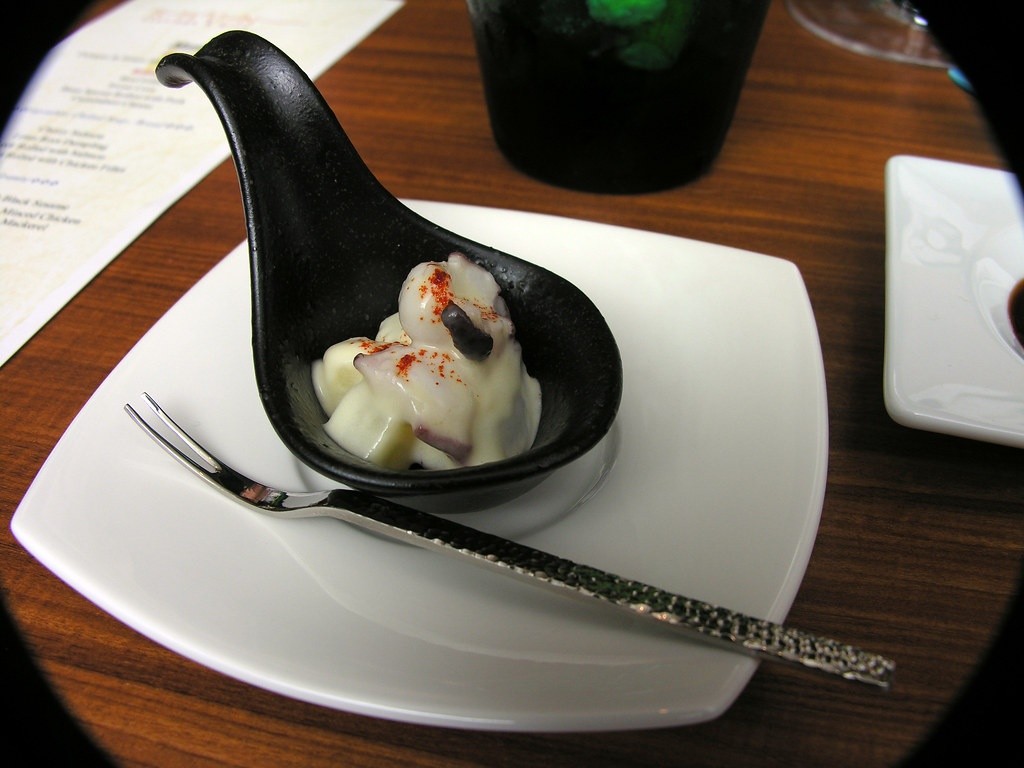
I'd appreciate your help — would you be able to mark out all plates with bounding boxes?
[9,194,830,734]
[882,154,1024,448]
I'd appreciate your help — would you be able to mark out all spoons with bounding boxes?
[154,27,623,516]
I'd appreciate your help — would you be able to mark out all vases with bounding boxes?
[464,0,771,196]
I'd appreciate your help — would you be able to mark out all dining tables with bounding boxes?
[0,1,1022,767]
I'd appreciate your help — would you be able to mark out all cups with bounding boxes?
[468,1,771,194]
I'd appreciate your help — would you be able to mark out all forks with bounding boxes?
[126,389,897,692]
